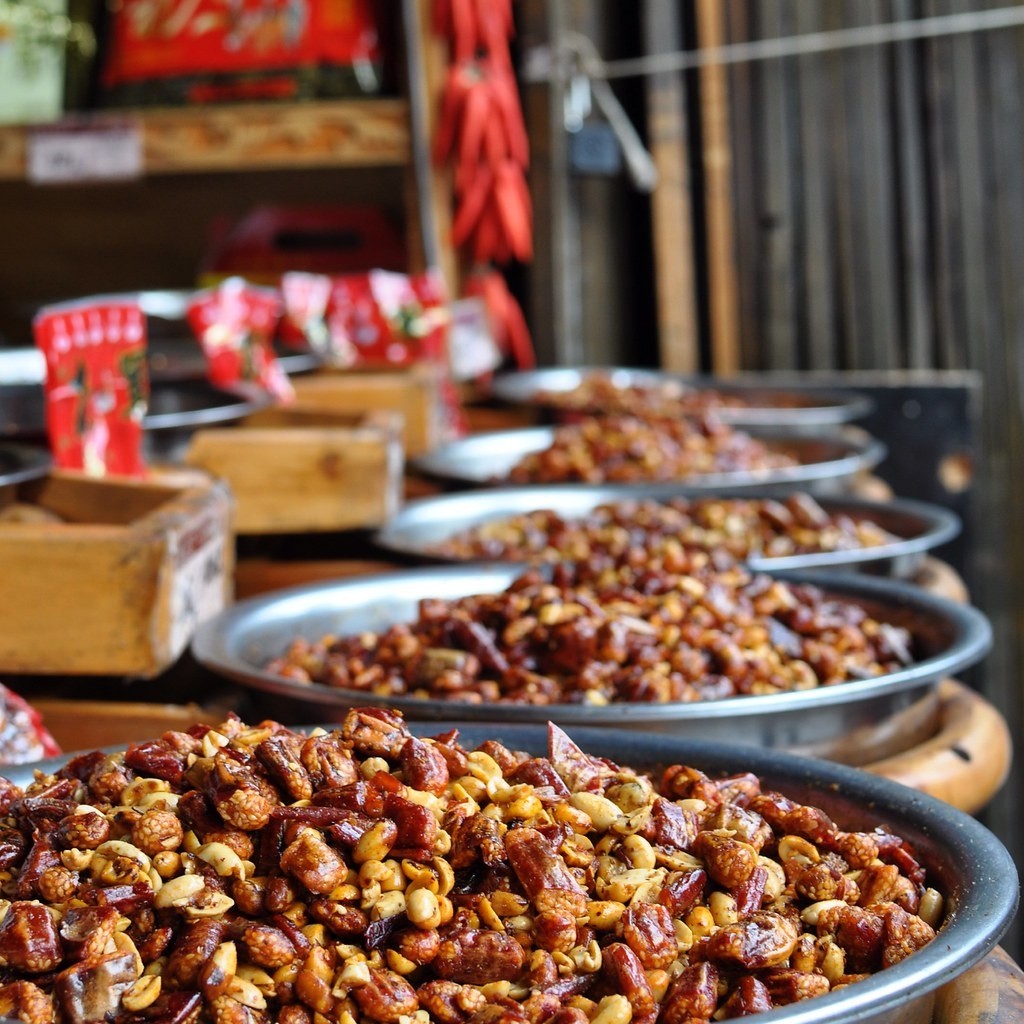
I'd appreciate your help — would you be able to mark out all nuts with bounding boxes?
[0,386,947,1024]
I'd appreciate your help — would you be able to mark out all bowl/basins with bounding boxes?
[0,281,1023,1023]
